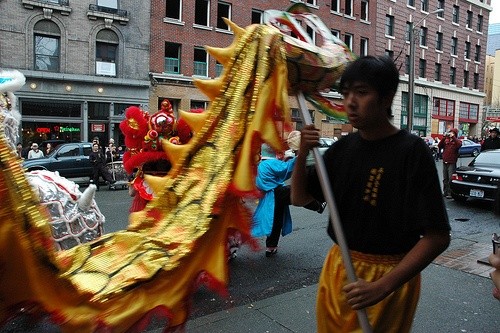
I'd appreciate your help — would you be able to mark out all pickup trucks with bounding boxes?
[21,142,124,184]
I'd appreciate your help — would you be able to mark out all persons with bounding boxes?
[290,55,452,333]
[14,125,500,256]
[488,254,500,301]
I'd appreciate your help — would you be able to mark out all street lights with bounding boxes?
[407,8,444,133]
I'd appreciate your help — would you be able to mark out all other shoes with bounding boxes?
[442,193,453,198]
[96,188,99,191]
[110,180,116,184]
[265,248,277,256]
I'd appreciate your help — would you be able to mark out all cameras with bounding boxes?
[446,133,450,136]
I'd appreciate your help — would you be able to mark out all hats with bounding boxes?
[31,143,39,149]
[489,127,500,135]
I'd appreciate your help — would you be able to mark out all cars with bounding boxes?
[283,137,335,164]
[448,148,500,208]
[458,139,481,157]
